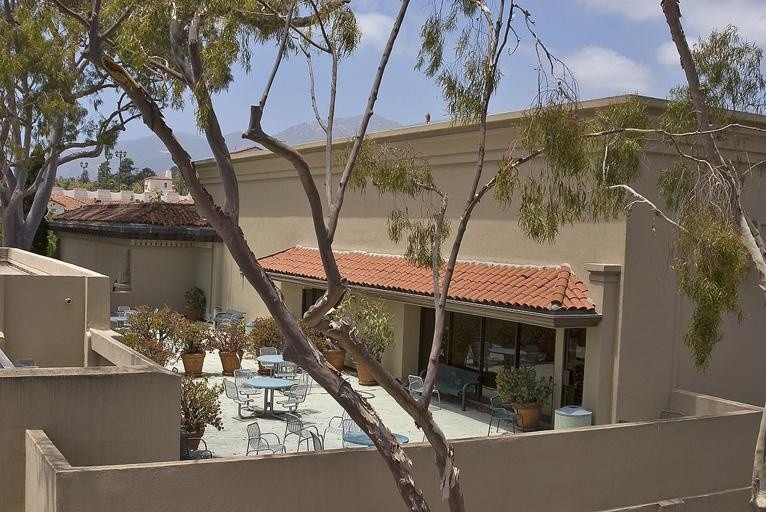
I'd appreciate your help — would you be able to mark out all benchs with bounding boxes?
[419,363,481,411]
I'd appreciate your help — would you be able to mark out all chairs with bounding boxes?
[180,438,212,460]
[488,395,524,436]
[223,347,441,456]
[471,341,539,372]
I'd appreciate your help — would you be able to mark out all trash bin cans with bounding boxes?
[554,406,593,431]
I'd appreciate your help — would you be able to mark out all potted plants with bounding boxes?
[181,376,226,452]
[117,287,396,386]
[490,366,556,428]
